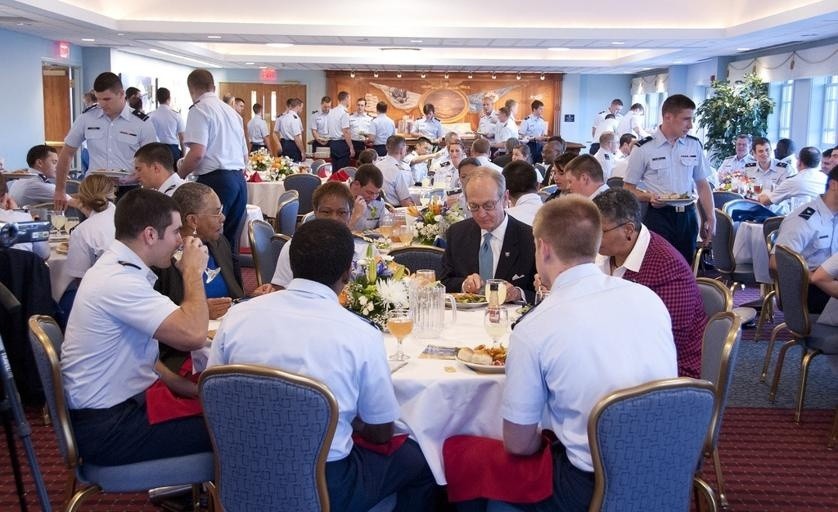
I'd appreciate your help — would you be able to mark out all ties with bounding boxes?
[478,232,493,281]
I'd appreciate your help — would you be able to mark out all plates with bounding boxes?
[445,293,488,309]
[655,198,696,207]
[455,353,505,372]
[1,173,38,179]
[88,170,130,178]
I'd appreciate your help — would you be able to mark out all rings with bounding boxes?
[467,284,470,289]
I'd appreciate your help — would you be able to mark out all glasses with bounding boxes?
[199,204,224,218]
[468,197,501,211]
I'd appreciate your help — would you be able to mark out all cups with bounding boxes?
[535,291,551,305]
[399,225,413,247]
[387,310,414,360]
[416,269,436,281]
[65,217,80,234]
[380,214,394,249]
[485,279,506,306]
[409,281,456,338]
[52,211,64,237]
[484,308,508,344]
[172,244,221,284]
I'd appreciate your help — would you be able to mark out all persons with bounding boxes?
[717,134,838,211]
[592,186,709,379]
[623,94,718,267]
[246,92,395,173]
[1,69,248,278]
[811,253,838,326]
[439,167,537,306]
[60,188,212,512]
[206,218,434,511]
[590,99,651,182]
[769,164,838,314]
[149,183,276,374]
[343,98,609,230]
[498,193,679,512]
[270,182,379,291]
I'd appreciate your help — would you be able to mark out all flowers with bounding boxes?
[249,146,271,167]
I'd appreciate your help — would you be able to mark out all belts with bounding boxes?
[659,203,695,213]
